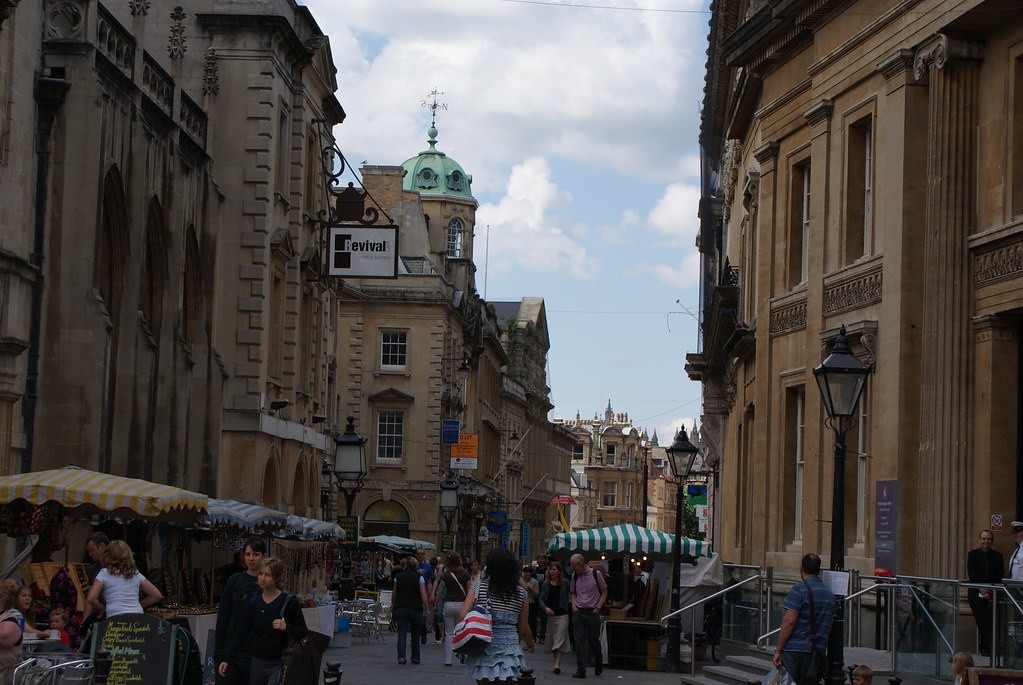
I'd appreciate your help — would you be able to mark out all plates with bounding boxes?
[22,638,39,641]
[45,638,62,641]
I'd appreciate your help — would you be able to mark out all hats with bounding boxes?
[1010,521,1023,533]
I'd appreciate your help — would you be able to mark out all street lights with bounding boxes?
[333,416,369,577]
[440,468,459,536]
[664,422,700,674]
[811,321,875,685]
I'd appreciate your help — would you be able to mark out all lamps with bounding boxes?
[809,322,876,458]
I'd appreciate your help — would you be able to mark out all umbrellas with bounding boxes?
[0,464,347,542]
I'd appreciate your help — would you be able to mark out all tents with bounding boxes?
[547,522,712,567]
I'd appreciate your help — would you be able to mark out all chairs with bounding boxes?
[7,654,95,685]
[331,599,398,646]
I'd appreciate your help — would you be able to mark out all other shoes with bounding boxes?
[553,666,561,674]
[595,653,603,676]
[572,669,586,678]
[421,633,427,644]
[411,656,420,663]
[436,636,445,645]
[539,639,544,644]
[399,659,407,664]
[532,639,536,643]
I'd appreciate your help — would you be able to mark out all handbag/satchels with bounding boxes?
[761,666,797,685]
[805,648,826,685]
[451,580,494,656]
[276,595,321,685]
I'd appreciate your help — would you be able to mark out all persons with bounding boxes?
[85,532,162,620]
[213,537,267,685]
[50,608,70,645]
[853,665,872,685]
[379,548,481,666]
[723,561,741,603]
[0,578,51,685]
[217,558,308,685]
[967,529,1005,657]
[773,553,836,685]
[520,554,547,645]
[951,652,974,685]
[569,554,608,679]
[539,562,572,674]
[1008,521,1023,630]
[459,549,536,685]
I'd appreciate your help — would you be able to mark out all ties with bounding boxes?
[1009,545,1020,579]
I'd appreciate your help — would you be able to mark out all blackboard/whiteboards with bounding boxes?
[202,628,218,685]
[90,613,177,685]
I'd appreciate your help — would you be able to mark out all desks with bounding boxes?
[21,638,62,661]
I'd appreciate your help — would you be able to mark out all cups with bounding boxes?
[49,630,61,638]
[22,633,29,638]
[29,633,37,638]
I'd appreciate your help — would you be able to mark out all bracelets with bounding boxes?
[595,607,600,611]
